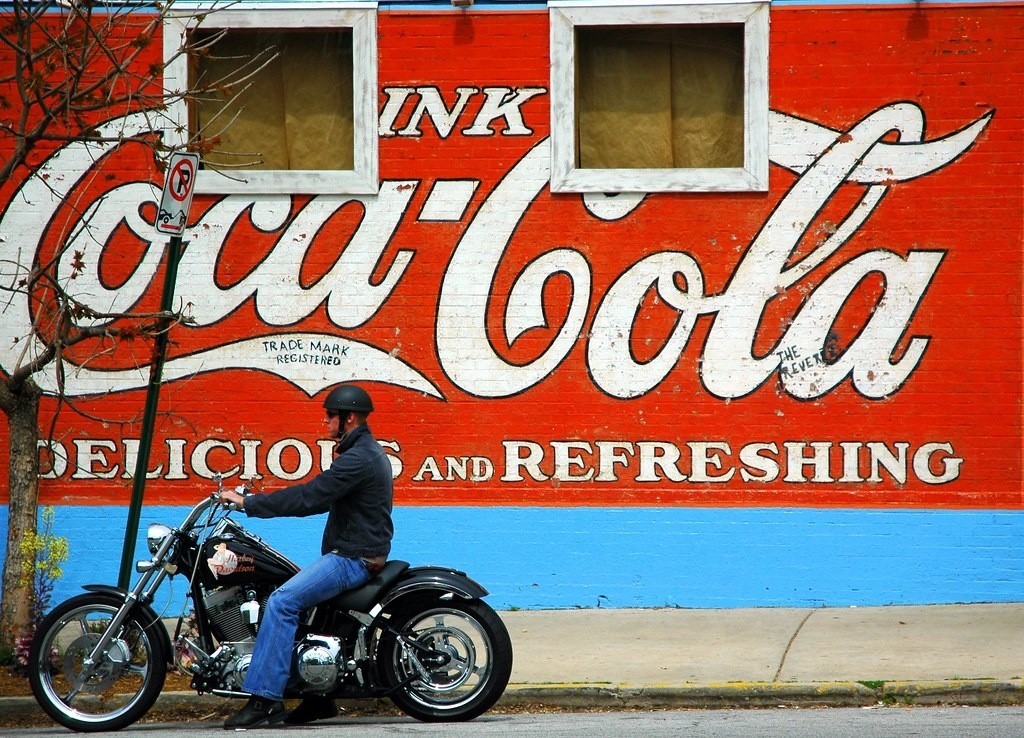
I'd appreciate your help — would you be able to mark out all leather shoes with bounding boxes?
[223,697,288,730]
[283,692,338,725]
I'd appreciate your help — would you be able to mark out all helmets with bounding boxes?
[323,385,375,412]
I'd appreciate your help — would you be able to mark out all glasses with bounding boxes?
[326,409,338,419]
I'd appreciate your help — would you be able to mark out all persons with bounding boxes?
[218,385,394,730]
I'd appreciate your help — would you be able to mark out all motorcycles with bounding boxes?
[24,471,513,734]
